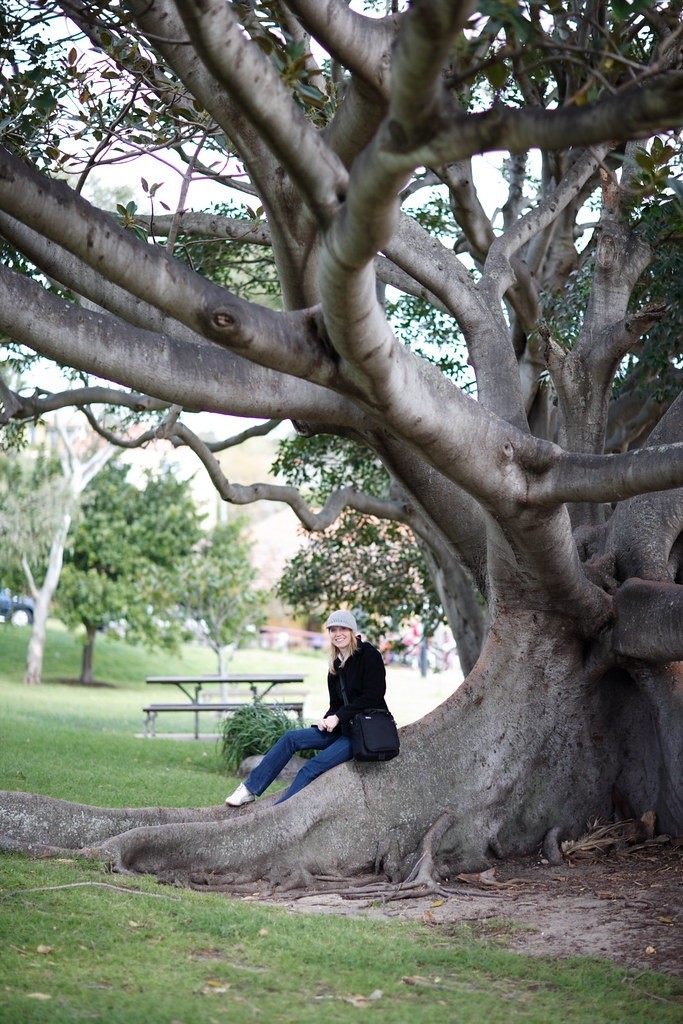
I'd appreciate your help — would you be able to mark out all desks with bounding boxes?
[147,674,305,740]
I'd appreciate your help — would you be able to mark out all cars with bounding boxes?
[0,586,36,628]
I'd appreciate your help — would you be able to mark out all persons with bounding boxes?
[225,609,387,811]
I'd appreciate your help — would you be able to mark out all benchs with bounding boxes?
[143,699,304,712]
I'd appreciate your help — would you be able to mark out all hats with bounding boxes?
[325,609,358,633]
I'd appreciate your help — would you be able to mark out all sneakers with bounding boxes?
[225,782,255,808]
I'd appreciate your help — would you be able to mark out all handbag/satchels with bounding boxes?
[350,707,401,761]
